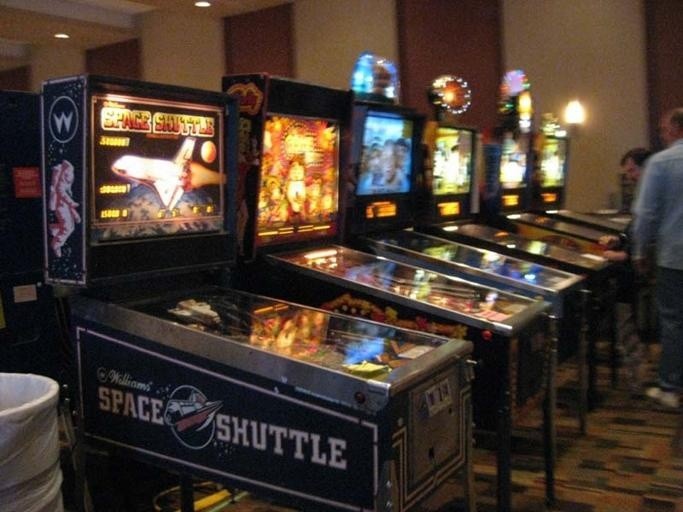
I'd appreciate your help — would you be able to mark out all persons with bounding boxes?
[629,108,682,409]
[356,138,411,193]
[598,148,664,403]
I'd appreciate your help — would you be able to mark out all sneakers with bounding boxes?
[645,387,681,409]
